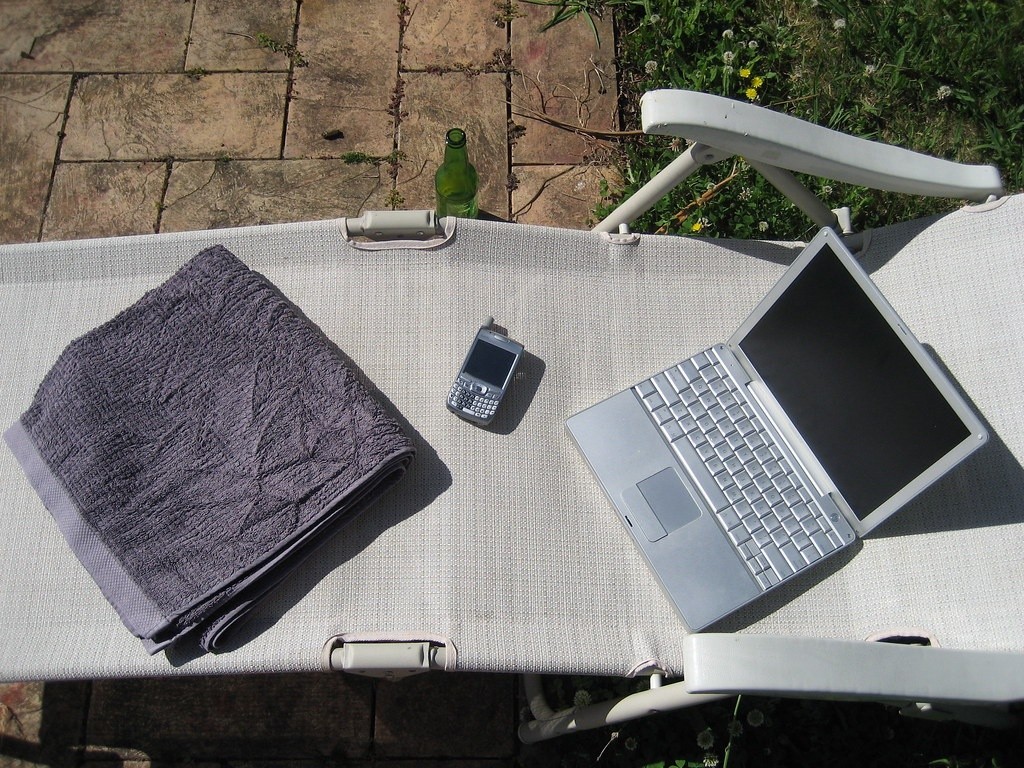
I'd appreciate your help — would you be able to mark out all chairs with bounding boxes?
[1,89,1024,745]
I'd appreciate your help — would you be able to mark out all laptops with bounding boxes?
[565,226,991,633]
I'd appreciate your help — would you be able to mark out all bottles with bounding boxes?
[434,128,479,219]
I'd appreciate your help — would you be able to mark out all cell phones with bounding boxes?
[446,316,524,426]
